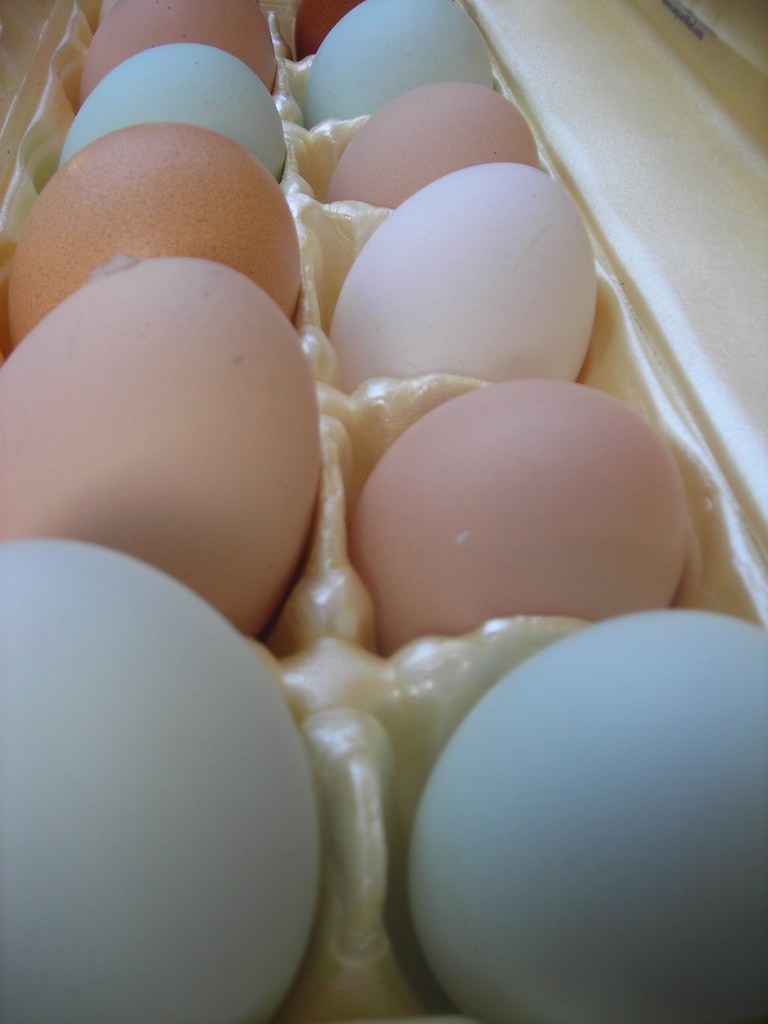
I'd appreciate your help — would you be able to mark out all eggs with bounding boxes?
[303,0,493,130]
[294,0,363,61]
[331,160,598,410]
[0,535,321,1024]
[79,0,276,110]
[58,43,285,186]
[327,83,537,208]
[351,381,688,652]
[8,119,300,353]
[407,610,768,1024]
[0,260,319,632]
